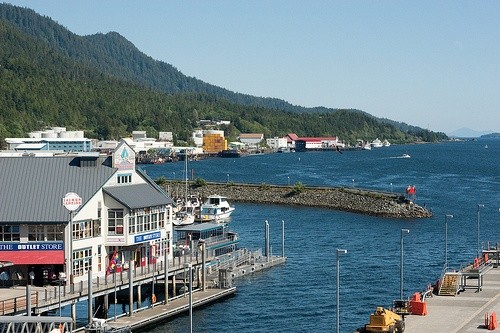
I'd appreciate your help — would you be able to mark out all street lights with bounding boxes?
[444,214,455,271]
[335,248,348,333]
[183,263,193,333]
[477,204,488,259]
[401,229,410,300]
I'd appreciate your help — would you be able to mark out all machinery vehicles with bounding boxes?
[360,306,405,333]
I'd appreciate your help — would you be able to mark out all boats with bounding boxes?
[172,192,236,228]
[370,138,384,148]
[384,139,390,147]
[403,154,410,158]
[362,141,372,150]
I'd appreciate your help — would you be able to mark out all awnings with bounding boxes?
[0,250,64,264]
